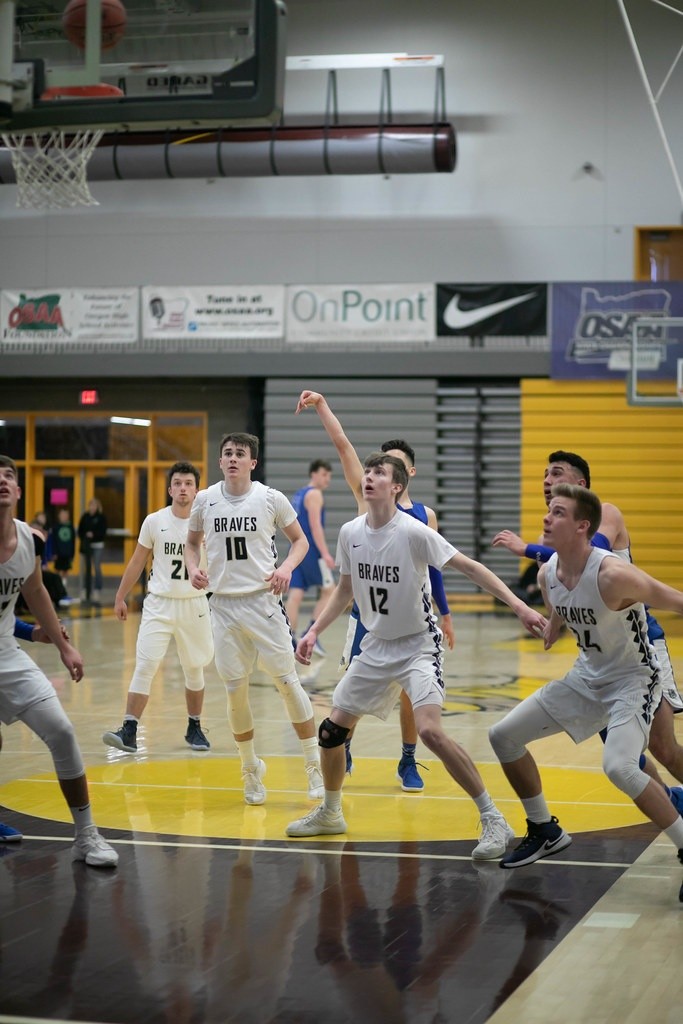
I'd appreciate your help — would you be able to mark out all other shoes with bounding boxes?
[672,787,683,817]
[300,630,327,657]
[0,823,22,841]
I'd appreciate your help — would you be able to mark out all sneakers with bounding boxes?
[397,760,424,792]
[71,825,118,868]
[305,760,325,799]
[104,728,137,752]
[241,759,266,805]
[677,848,683,902]
[345,755,353,772]
[499,816,573,869]
[285,801,346,836]
[472,811,514,859]
[185,726,210,750]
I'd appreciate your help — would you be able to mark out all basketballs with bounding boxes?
[62,0,128,52]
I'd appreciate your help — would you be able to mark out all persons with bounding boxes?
[490,476,683,909]
[180,432,327,804]
[284,452,547,859]
[31,511,50,568]
[76,497,109,603]
[102,462,214,751]
[284,459,338,659]
[49,509,79,579]
[293,390,457,795]
[0,448,120,868]
[491,449,683,812]
